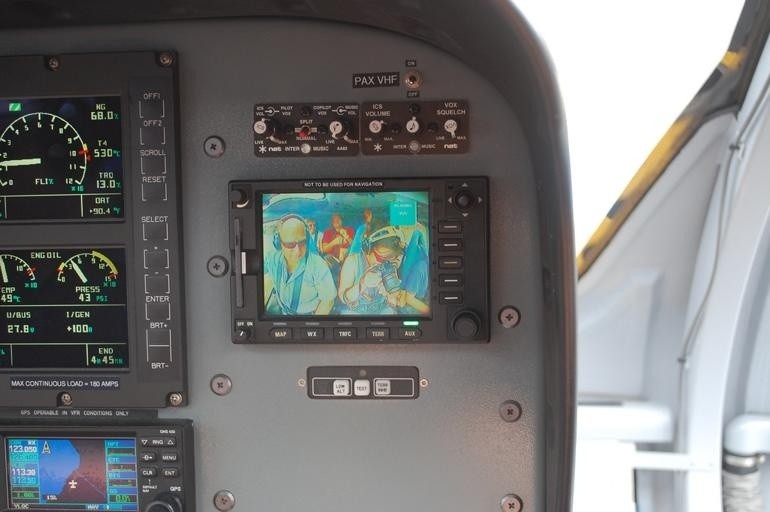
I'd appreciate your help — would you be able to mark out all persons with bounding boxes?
[263,210,430,314]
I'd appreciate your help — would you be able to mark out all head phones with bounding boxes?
[273,214,310,251]
[361,226,402,255]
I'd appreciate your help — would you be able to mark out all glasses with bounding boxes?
[373,250,399,262]
[280,240,306,248]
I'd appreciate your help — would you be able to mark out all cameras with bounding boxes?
[371,260,401,292]
